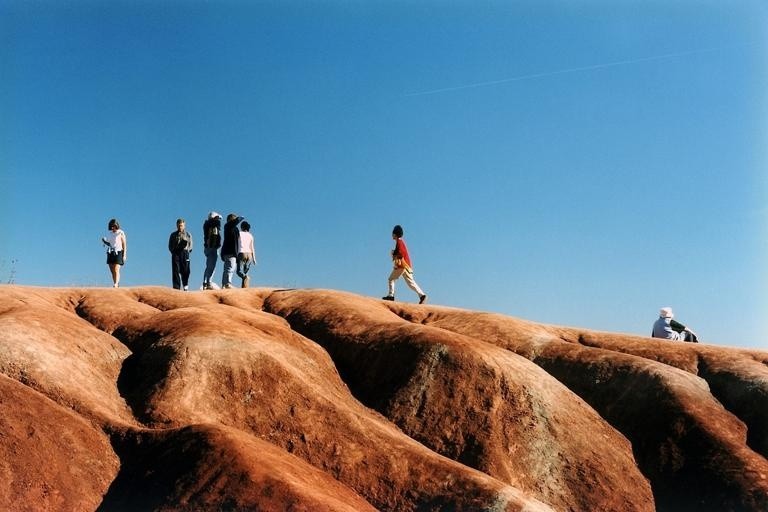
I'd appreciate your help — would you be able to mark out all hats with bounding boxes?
[660,308,672,318]
[208,211,218,218]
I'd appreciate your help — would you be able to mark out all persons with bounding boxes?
[203,211,223,289]
[103,219,126,287]
[221,215,244,288]
[169,219,192,291]
[652,307,699,342]
[382,225,426,304]
[236,221,257,288]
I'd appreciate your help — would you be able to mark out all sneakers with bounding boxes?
[113,283,119,287]
[383,296,394,301]
[184,284,188,290]
[419,295,426,303]
[202,276,249,289]
